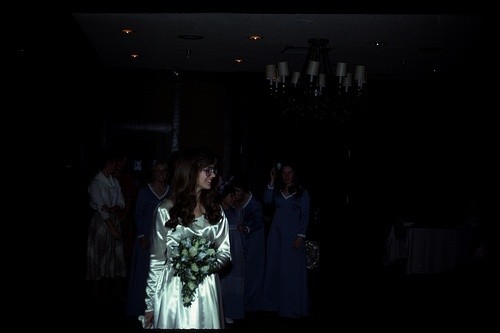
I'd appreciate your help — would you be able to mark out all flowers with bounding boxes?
[170,236,223,308]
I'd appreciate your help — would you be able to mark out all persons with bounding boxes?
[86,146,129,298]
[145,150,231,330]
[217,183,245,324]
[127,159,170,315]
[234,185,265,311]
[264,162,310,318]
[119,190,134,257]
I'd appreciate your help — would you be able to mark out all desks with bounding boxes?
[404,228,460,275]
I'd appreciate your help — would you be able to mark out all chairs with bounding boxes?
[383,225,409,268]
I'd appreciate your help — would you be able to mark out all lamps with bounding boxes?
[264,39,368,122]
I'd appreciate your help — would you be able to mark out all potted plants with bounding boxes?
[488,246,497,280]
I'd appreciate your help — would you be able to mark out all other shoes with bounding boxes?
[225,317,234,323]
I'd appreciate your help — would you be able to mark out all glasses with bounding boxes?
[203,166,218,173]
